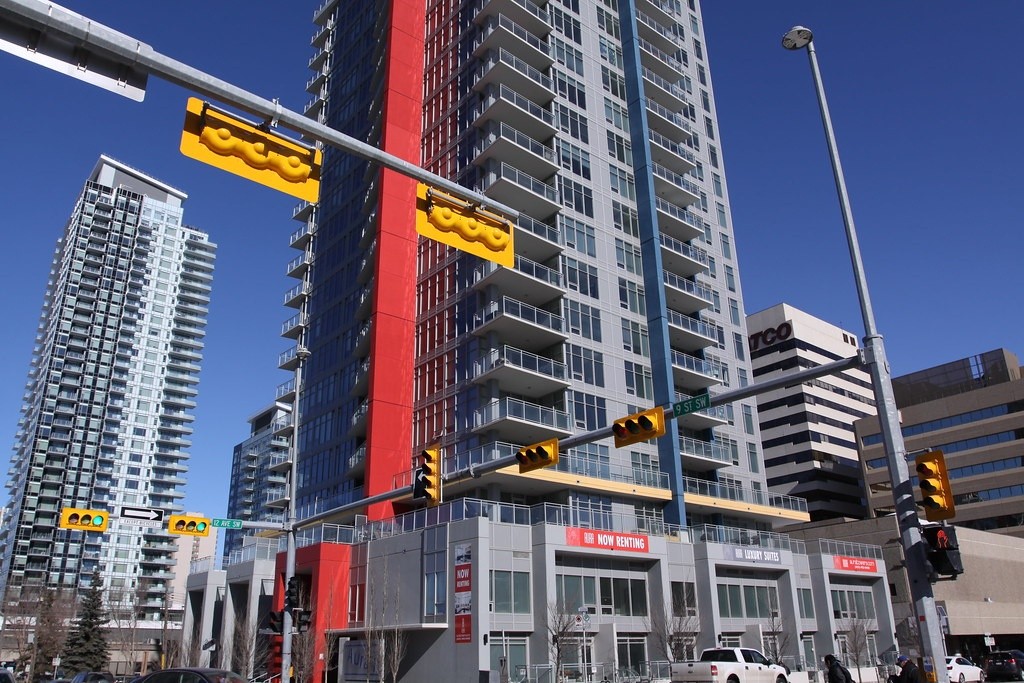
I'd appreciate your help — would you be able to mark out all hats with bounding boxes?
[895,656,907,665]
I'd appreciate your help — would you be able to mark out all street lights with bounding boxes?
[781,24,953,683]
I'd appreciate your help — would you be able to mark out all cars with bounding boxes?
[945,656,986,683]
[133,666,252,683]
[0,667,18,683]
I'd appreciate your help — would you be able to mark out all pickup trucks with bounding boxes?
[41,671,116,683]
[668,645,791,683]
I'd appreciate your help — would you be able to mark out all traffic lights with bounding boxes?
[923,525,958,551]
[268,610,284,632]
[168,514,210,537]
[296,610,312,633]
[421,441,439,509]
[611,406,666,449]
[282,576,300,607]
[516,437,559,474]
[914,449,958,521]
[60,507,109,532]
[926,550,963,576]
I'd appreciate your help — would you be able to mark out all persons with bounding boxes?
[889,656,925,683]
[825,654,856,683]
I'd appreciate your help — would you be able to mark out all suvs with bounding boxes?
[987,648,1024,681]
